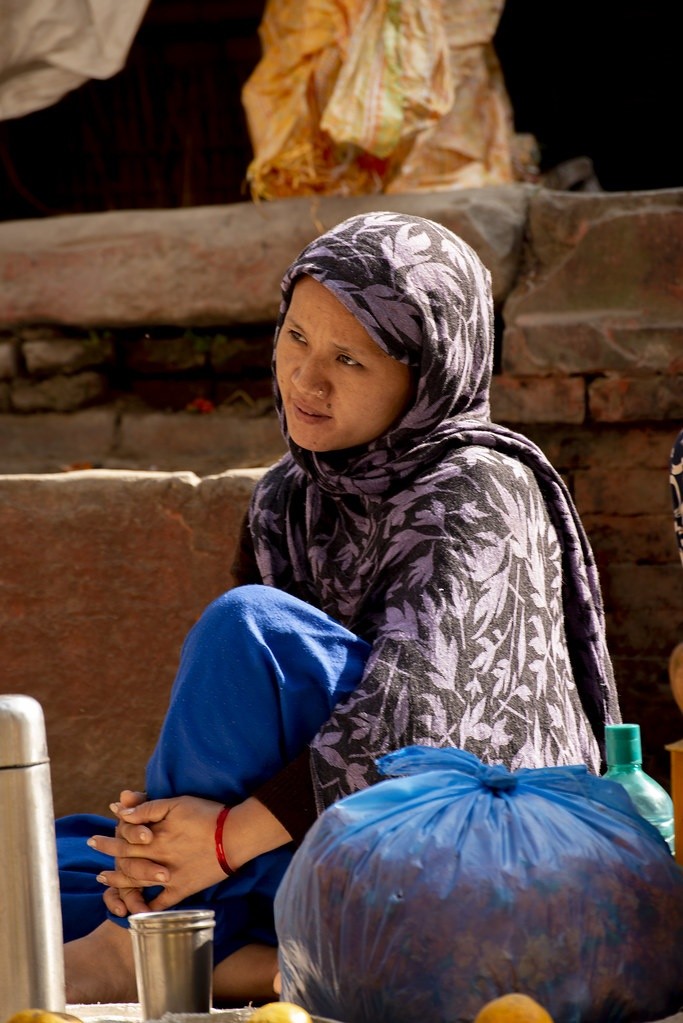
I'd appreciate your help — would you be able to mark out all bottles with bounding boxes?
[600,724,675,857]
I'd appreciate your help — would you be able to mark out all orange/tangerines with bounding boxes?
[474,993,553,1023]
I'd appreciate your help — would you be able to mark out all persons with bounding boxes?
[56,208,622,999]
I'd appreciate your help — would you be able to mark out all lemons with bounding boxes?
[248,1001,312,1023]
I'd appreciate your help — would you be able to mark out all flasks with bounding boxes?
[0,692,68,1022]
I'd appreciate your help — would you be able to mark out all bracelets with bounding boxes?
[215,803,235,880]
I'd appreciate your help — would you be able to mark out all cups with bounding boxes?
[127,909,216,1021]
